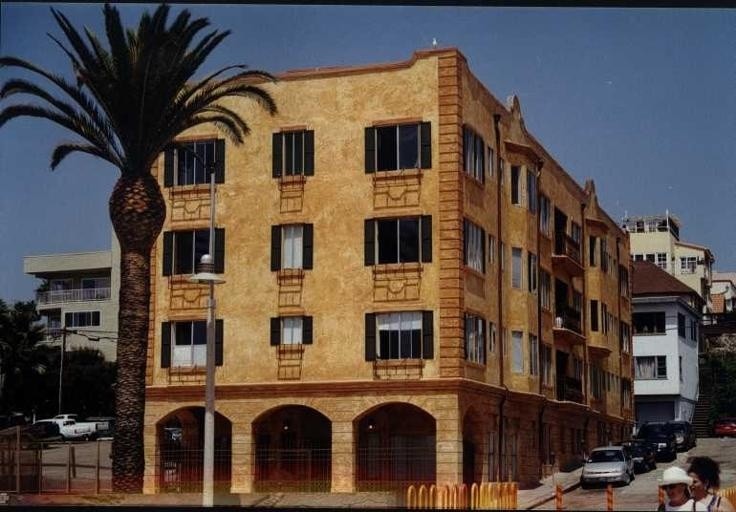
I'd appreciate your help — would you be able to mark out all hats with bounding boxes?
[658,467,693,487]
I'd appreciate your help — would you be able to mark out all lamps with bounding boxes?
[281,417,290,435]
[368,418,377,433]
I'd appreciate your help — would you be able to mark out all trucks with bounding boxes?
[0,413,110,450]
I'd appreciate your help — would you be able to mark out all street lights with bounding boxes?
[185,252,225,510]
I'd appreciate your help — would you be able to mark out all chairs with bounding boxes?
[540,462,559,489]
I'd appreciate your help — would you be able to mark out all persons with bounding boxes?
[686,456,735,511]
[656,466,706,511]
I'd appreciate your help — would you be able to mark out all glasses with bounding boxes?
[662,484,680,489]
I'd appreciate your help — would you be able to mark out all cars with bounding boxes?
[670,420,696,452]
[615,438,655,475]
[579,446,635,490]
[712,417,735,438]
[632,421,676,462]
